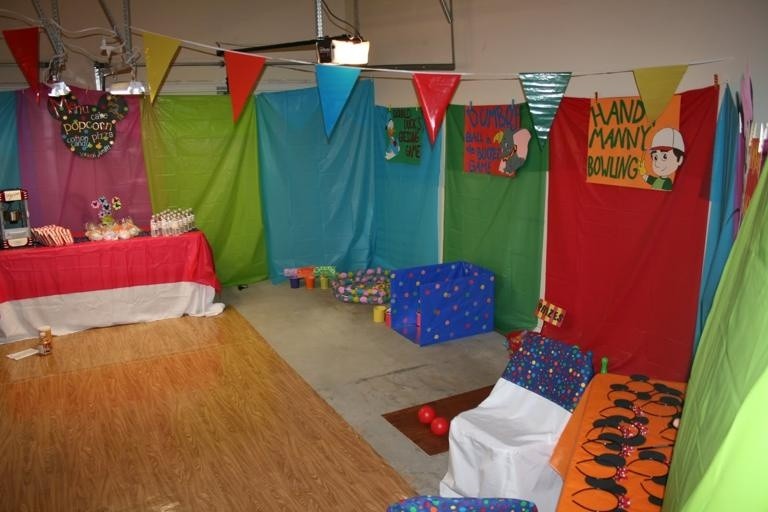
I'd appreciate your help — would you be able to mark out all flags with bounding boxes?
[315,64,361,140]
[224,52,266,123]
[2,27,40,91]
[517,71,572,151]
[143,32,181,103]
[633,65,689,121]
[411,74,461,144]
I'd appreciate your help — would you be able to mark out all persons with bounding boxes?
[638,127,685,191]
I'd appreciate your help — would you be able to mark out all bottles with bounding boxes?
[149,207,196,237]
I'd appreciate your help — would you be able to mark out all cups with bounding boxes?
[290,278,300,288]
[320,278,329,289]
[305,277,315,288]
[373,306,386,322]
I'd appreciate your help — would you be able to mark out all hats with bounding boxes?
[649,127,687,153]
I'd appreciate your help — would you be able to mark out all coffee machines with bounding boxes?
[0,190,32,249]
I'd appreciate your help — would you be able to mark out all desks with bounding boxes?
[0,224,225,345]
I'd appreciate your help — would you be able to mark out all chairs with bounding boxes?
[439,330,592,512]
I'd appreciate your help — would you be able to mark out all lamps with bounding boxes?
[316,1,371,66]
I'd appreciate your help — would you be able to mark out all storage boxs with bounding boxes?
[389,261,496,348]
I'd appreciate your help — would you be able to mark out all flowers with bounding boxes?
[91,195,121,218]
